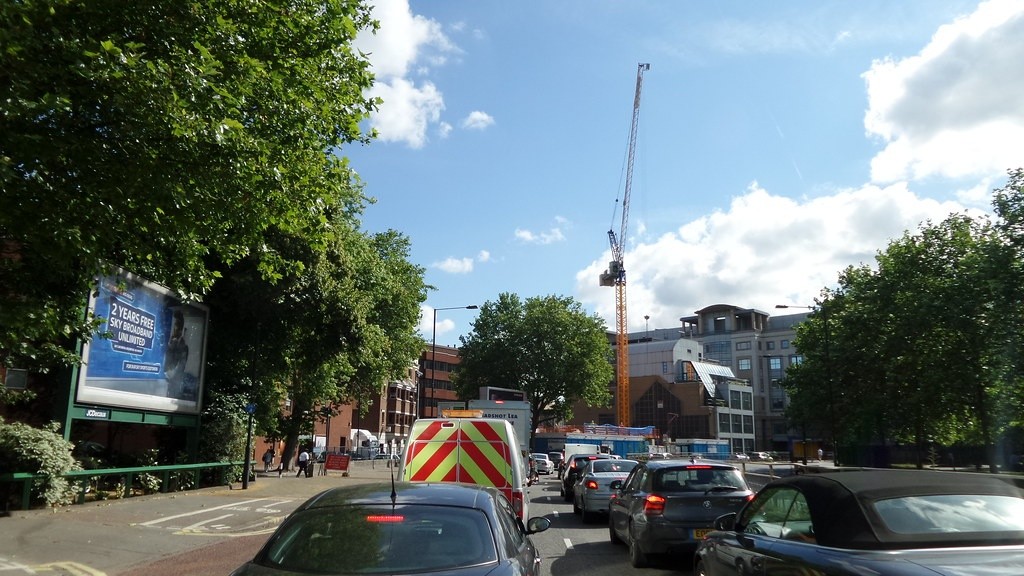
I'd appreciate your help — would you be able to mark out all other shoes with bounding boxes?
[266,470,267,472]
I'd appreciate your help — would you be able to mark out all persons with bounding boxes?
[817,448,823,461]
[164,313,188,400]
[296,449,309,478]
[262,446,275,473]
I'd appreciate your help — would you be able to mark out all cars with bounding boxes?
[608,460,757,568]
[526,451,705,502]
[572,458,650,525]
[730,452,750,463]
[692,469,1024,576]
[227,480,551,576]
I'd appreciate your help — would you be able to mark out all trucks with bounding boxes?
[436,401,484,419]
[351,428,379,457]
[563,443,602,470]
[467,398,533,480]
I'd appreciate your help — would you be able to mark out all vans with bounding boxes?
[751,452,773,463]
[395,409,535,534]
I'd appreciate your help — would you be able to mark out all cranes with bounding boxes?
[598,61,651,424]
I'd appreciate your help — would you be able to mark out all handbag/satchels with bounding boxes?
[262,453,266,461]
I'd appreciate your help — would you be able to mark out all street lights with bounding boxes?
[775,304,840,467]
[430,305,478,415]
